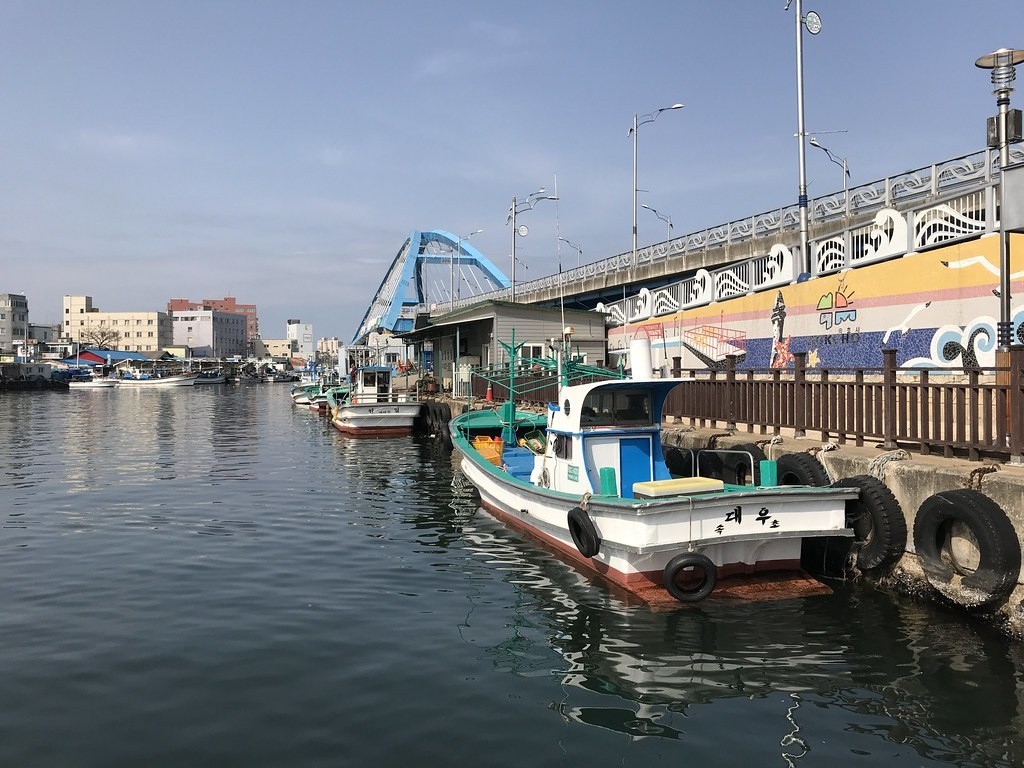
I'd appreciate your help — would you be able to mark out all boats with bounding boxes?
[92,371,201,386]
[448,325,861,613]
[326,364,425,440]
[290,362,341,412]
[69,378,119,387]
[195,370,292,384]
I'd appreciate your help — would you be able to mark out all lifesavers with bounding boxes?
[664,552,717,604]
[568,508,600,559]
[437,424,452,455]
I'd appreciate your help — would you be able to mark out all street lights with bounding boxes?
[455,229,483,300]
[557,236,583,267]
[506,190,560,302]
[419,245,454,312]
[627,103,686,267]
[809,137,851,199]
[975,48,1023,447]
[508,254,528,282]
[641,204,673,241]
